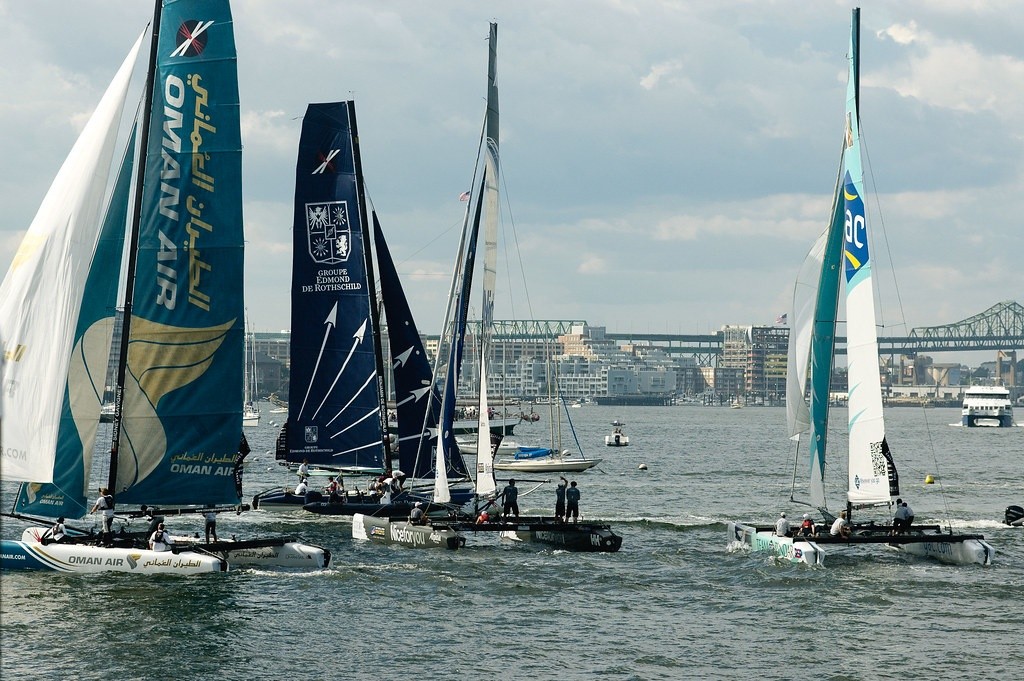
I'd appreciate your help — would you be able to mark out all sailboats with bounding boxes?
[100,370,116,423]
[243,306,261,428]
[728,8,997,571]
[0,1,331,575]
[253,21,623,552]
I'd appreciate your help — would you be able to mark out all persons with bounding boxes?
[299,459,310,486]
[892,498,914,536]
[615,428,623,435]
[501,479,519,516]
[295,479,310,495]
[91,488,114,532]
[411,501,431,526]
[554,476,568,517]
[565,481,580,522]
[52,517,87,545]
[202,506,220,544]
[141,505,164,531]
[465,407,491,417]
[320,476,343,494]
[830,512,847,538]
[149,523,176,552]
[776,512,793,537]
[369,470,406,493]
[798,513,815,537]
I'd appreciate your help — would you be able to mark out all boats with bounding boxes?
[572,397,598,408]
[962,385,1013,428]
[604,420,629,446]
[528,397,563,405]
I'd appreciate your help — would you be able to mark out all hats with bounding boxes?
[481,511,487,515]
[302,479,308,482]
[415,502,423,506]
[558,483,563,487]
[781,512,786,517]
[803,514,810,520]
